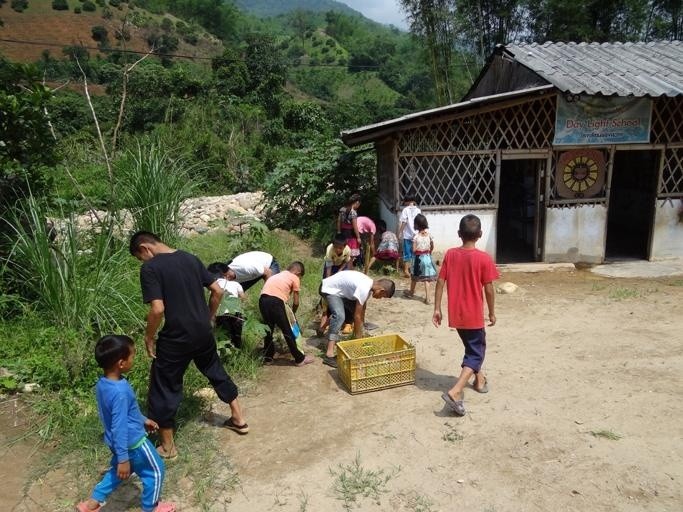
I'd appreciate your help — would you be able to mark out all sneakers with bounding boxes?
[322,355,338,368]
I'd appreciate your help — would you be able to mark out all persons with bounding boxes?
[128,230,248,459]
[70,335,180,512]
[203,262,250,365]
[314,193,438,369]
[206,250,281,297]
[429,213,496,416]
[257,261,315,366]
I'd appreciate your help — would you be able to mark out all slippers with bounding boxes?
[425,298,431,305]
[474,377,488,393]
[158,454,178,461]
[442,392,465,416]
[76,499,102,512]
[296,355,315,365]
[154,500,175,512]
[403,288,414,297]
[224,415,249,434]
